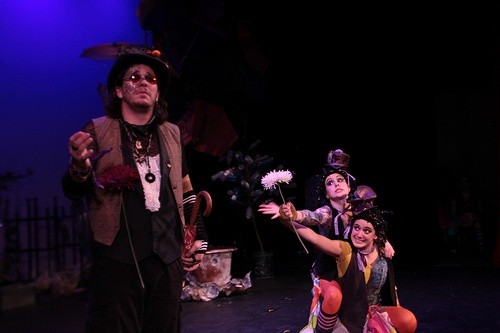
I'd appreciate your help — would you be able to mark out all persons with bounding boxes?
[259,201,417,333]
[276,148,371,333]
[210,140,274,248]
[63,48,206,332]
[179,109,215,193]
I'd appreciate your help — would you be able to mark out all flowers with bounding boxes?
[96,164,148,289]
[261,169,310,254]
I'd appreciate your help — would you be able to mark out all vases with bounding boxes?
[191,245,239,285]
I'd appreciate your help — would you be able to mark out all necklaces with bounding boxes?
[118,120,157,183]
[357,243,376,254]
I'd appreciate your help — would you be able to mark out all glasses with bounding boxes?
[123,74,159,83]
